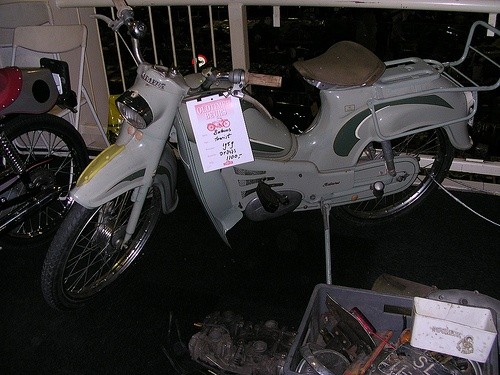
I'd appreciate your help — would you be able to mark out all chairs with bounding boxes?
[0,0,112,200]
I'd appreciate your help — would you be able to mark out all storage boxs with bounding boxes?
[284,283,500,375]
[409,297,496,363]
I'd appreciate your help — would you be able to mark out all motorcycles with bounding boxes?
[40,0,500,317]
[1,57,92,251]
